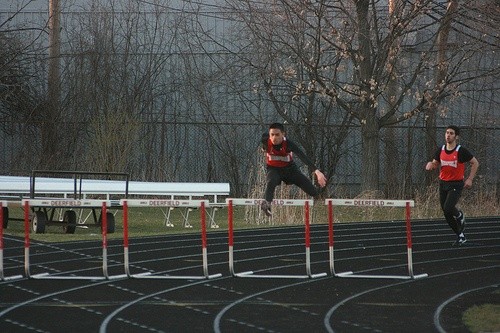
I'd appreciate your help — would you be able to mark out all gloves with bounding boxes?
[261,133,269,150]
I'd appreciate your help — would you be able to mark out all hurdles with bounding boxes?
[22,197,127,281]
[325,198,429,281]
[0,199,23,282]
[118,198,223,279]
[226,197,327,279]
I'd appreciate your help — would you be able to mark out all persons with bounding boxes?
[259,123,328,217]
[425,124,480,247]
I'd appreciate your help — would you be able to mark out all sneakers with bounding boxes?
[454,210,464,225]
[261,201,272,215]
[452,235,468,247]
